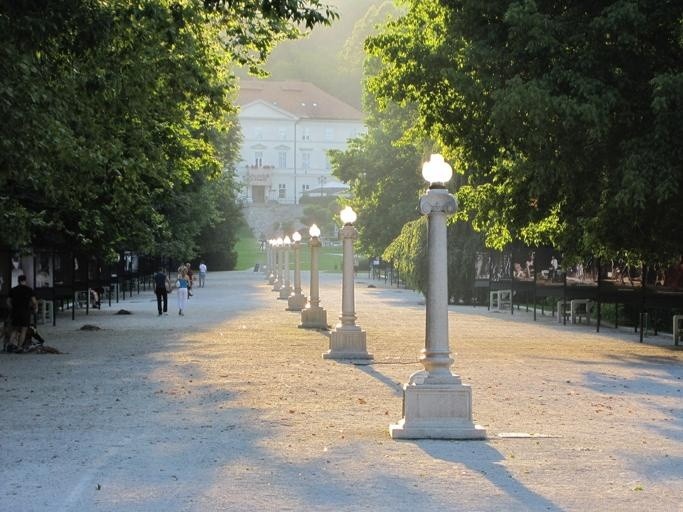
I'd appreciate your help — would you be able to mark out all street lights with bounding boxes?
[306,223,322,308]
[415,152,458,370]
[265,235,290,287]
[291,232,303,293]
[336,205,360,324]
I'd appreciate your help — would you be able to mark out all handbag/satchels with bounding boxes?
[176,281,180,288]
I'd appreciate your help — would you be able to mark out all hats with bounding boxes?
[19,275,26,281]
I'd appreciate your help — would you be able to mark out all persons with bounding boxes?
[174,265,190,317]
[185,262,193,297]
[0,274,45,351]
[548,254,558,280]
[150,266,172,316]
[197,260,206,288]
[88,278,104,309]
[510,261,526,297]
